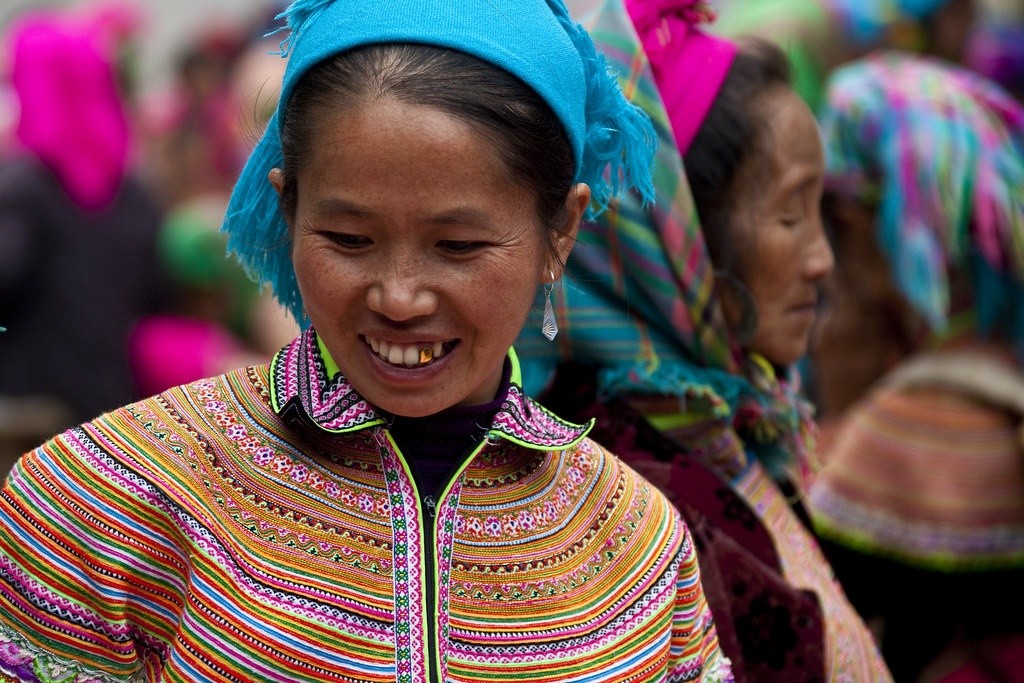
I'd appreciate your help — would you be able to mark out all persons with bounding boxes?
[0,0,301,424]
[0,0,735,683]
[536,0,895,683]
[802,50,1024,683]
